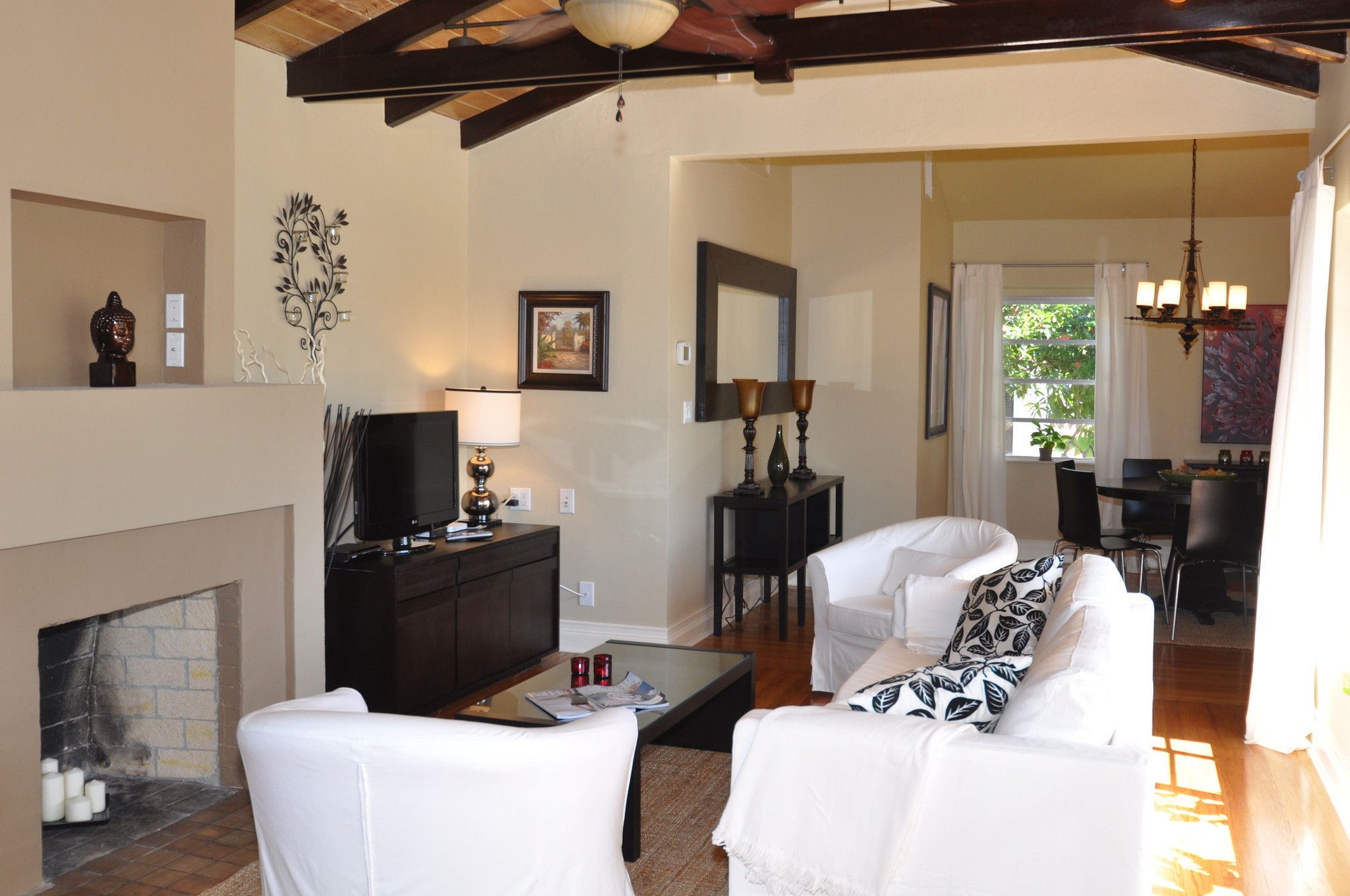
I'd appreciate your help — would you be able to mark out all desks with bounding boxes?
[713,475,845,641]
[1094,477,1255,626]
[1183,460,1260,474]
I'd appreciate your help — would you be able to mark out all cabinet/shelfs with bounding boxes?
[324,523,560,714]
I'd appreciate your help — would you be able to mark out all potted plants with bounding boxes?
[1029,420,1072,461]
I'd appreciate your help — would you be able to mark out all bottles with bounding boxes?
[767,425,790,486]
[1218,449,1232,464]
[1240,450,1254,467]
[1259,451,1270,469]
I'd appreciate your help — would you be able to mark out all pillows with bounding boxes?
[938,555,1062,664]
[847,655,1032,733]
[882,547,970,596]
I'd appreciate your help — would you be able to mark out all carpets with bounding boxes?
[198,745,730,896]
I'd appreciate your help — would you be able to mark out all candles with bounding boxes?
[41,758,106,822]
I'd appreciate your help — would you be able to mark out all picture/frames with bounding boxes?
[925,282,952,440]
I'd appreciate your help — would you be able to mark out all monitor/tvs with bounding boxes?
[351,411,459,554]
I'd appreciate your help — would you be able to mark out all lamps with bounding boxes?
[736,381,766,490]
[274,191,351,365]
[1125,139,1257,357]
[445,386,521,527]
[561,0,681,123]
[790,380,816,474]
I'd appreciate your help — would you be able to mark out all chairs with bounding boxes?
[1055,458,1257,640]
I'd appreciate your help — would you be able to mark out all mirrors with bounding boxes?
[695,241,798,423]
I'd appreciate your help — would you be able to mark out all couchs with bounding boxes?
[807,516,1018,693]
[710,554,1154,896]
[235,687,638,896]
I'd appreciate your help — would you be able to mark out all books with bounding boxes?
[525,669,670,720]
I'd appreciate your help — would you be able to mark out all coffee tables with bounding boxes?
[457,640,756,863]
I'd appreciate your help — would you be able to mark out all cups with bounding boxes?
[593,654,612,680]
[571,657,589,675]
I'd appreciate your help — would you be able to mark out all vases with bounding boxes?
[767,425,790,486]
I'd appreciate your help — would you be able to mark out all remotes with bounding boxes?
[444,531,493,542]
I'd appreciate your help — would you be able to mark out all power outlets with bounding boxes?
[510,488,531,510]
[579,582,593,606]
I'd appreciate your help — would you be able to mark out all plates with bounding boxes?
[1157,470,1238,485]
